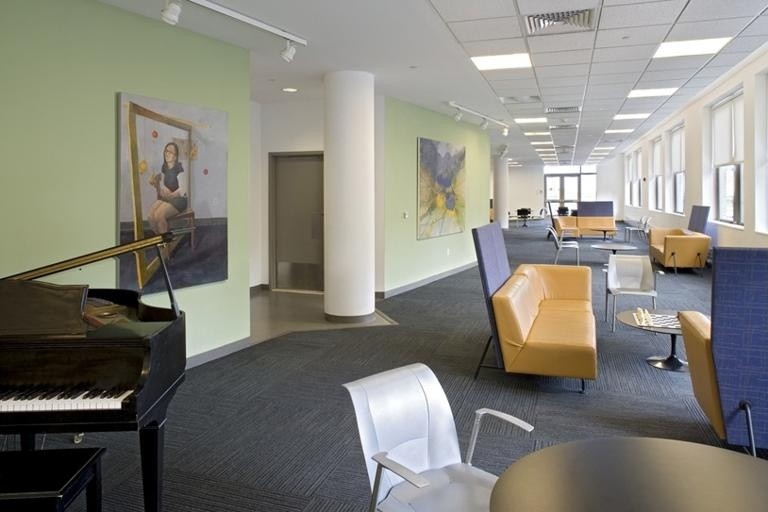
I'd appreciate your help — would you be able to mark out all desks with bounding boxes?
[509,216,544,228]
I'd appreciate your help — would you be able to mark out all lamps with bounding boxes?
[480,119,490,130]
[502,126,510,135]
[158,0,182,25]
[280,39,295,62]
[453,110,462,122]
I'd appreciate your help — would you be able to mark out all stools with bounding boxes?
[0,444,108,512]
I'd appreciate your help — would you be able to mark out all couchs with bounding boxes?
[473,223,599,393]
[546,201,580,241]
[648,205,711,279]
[677,246,768,451]
[577,201,615,240]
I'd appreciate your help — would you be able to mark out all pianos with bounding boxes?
[0,232,188,511]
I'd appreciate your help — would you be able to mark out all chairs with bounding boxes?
[624,216,645,243]
[628,216,652,242]
[545,226,580,266]
[604,254,659,334]
[342,363,535,512]
[547,225,578,259]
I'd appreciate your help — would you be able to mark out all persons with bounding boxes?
[148,143,188,243]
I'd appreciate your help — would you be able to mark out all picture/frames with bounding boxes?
[116,92,228,298]
